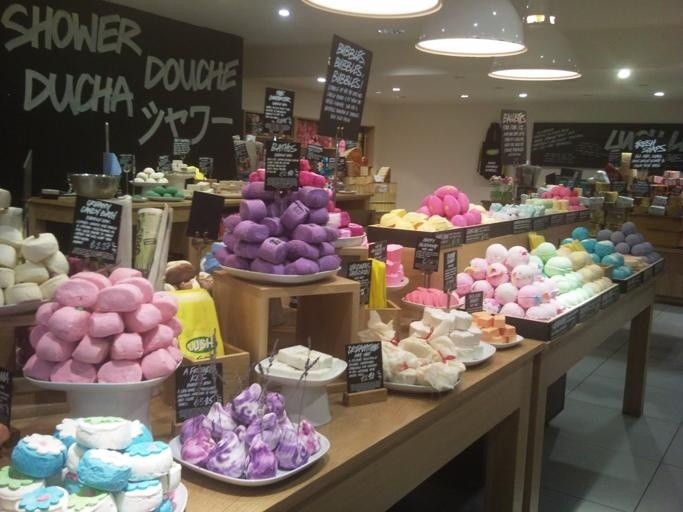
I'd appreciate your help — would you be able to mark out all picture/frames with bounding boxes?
[220,192,375,234]
[0,311,36,369]
[27,196,195,256]
[602,210,682,308]
[180,334,543,511]
[359,218,600,303]
[520,273,665,511]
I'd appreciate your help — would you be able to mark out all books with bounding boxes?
[182,343,250,409]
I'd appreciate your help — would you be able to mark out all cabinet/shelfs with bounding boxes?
[336,124,347,156]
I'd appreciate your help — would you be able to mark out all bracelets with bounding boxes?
[67,172,121,199]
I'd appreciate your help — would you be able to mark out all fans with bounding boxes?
[530,122,683,171]
[315,32,373,141]
[501,109,528,166]
[0,0,243,207]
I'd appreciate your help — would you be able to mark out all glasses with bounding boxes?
[415,1,528,58]
[303,0,442,17]
[486,0,582,80]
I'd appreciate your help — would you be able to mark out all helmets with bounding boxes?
[0,297,57,318]
[382,332,525,395]
[165,430,331,486]
[339,191,357,195]
[172,481,188,512]
[222,262,342,288]
[133,196,186,202]
